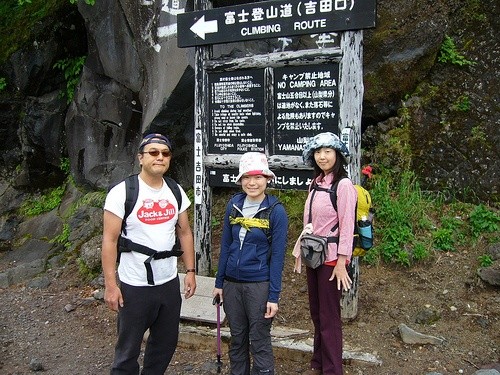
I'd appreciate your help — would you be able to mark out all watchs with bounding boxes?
[184,269,195,274]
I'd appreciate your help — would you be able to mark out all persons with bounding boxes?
[292,132,359,375]
[212,152,289,375]
[101,133,197,375]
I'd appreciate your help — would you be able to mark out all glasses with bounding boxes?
[140,150,171,157]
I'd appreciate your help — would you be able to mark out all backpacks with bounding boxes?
[310,178,373,257]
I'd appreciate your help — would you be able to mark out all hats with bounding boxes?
[235,152,275,186]
[302,131,350,166]
[139,133,172,149]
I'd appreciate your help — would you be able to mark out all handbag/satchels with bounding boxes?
[300,234,327,268]
